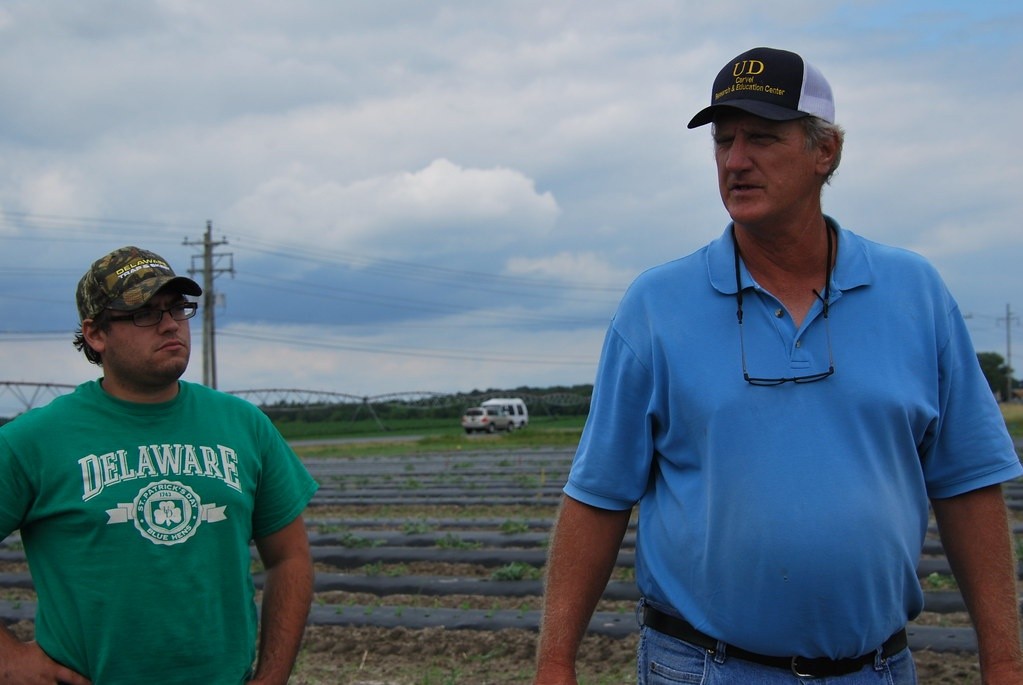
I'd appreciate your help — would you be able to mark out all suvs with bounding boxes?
[462,406,516,436]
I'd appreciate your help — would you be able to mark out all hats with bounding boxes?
[687,47,836,129]
[76,246,202,325]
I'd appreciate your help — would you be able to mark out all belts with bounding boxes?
[642,605,908,677]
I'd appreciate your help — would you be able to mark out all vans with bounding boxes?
[480,395,530,432]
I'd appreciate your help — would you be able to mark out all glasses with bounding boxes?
[94,301,198,333]
[729,286,835,387]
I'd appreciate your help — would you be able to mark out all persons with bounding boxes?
[1,245,319,685]
[533,45,1023,685]
